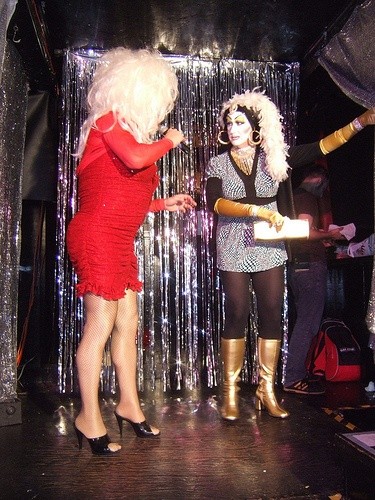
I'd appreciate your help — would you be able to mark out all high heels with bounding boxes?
[113,408,160,437]
[72,421,122,455]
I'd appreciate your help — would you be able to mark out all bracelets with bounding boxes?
[352,118,364,131]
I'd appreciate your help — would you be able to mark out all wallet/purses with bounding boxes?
[253,219,309,242]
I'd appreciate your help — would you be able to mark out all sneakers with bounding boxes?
[283,373,327,394]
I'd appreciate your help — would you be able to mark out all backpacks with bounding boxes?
[305,318,362,383]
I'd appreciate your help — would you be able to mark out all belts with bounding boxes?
[292,254,320,262]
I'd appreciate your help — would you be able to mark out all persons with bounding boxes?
[65,47,197,455]
[281,163,346,394]
[205,93,375,422]
[335,229,375,391]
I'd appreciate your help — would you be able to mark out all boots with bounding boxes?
[255,338,290,419]
[219,337,246,421]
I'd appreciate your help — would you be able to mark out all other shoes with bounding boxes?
[358,401,375,409]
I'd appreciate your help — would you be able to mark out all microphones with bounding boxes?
[159,125,191,154]
